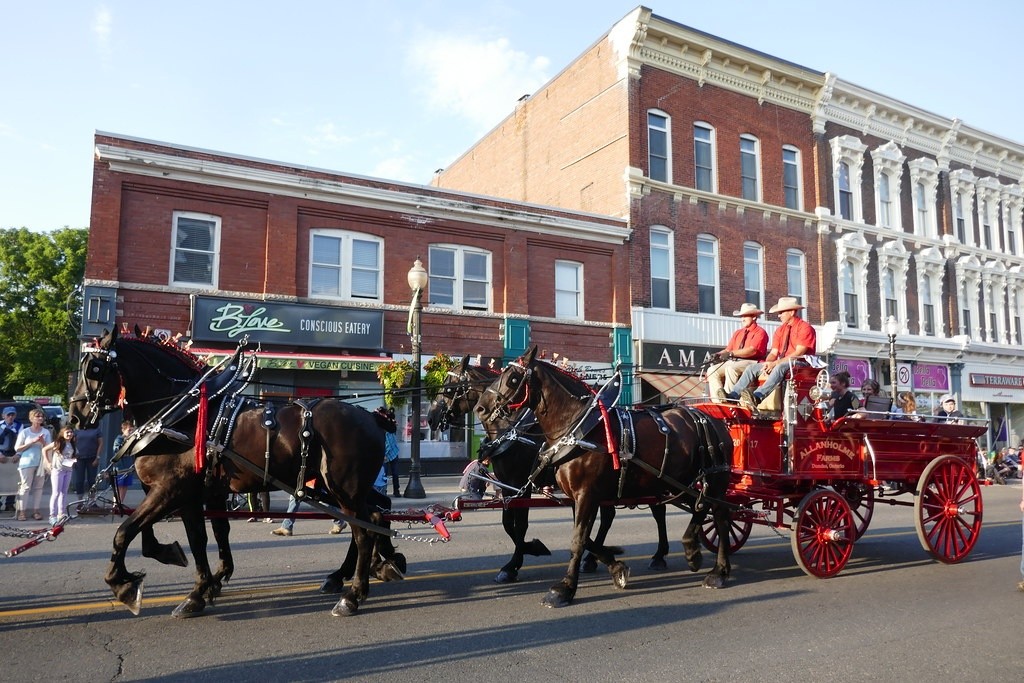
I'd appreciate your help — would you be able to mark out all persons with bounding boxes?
[891,391,926,423]
[41,426,79,525]
[976,445,1024,592]
[810,372,897,428]
[372,432,400,496]
[0,407,23,511]
[458,456,502,507]
[273,493,347,536]
[932,394,965,425]
[113,419,136,508]
[14,408,51,521]
[707,303,769,403]
[246,491,274,524]
[717,297,816,416]
[72,428,104,510]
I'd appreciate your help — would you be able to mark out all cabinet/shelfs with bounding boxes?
[401,398,430,441]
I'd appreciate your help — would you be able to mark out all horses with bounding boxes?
[471,345,734,607]
[426,353,624,584]
[70,325,406,619]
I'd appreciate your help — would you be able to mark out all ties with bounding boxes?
[782,326,791,358]
[737,330,750,349]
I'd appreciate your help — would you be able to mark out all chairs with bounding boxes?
[0,457,21,519]
[864,395,893,419]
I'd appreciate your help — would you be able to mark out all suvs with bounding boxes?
[0,398,55,438]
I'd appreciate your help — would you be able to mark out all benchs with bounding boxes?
[746,358,824,413]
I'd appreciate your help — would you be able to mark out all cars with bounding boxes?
[41,404,69,429]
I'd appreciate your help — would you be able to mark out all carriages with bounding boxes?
[68,320,992,619]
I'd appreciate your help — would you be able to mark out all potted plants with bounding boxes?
[377,357,417,410]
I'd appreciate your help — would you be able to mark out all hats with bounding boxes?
[733,303,764,316]
[3,407,17,414]
[940,394,955,403]
[769,297,806,313]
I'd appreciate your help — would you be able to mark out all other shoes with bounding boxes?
[262,517,273,523]
[329,523,348,534]
[32,511,43,520]
[390,490,402,497]
[742,387,760,407]
[271,527,292,537]
[718,388,734,403]
[49,516,63,525]
[18,512,26,521]
[247,517,257,522]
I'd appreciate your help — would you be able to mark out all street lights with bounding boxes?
[402,255,430,498]
[886,315,899,406]
